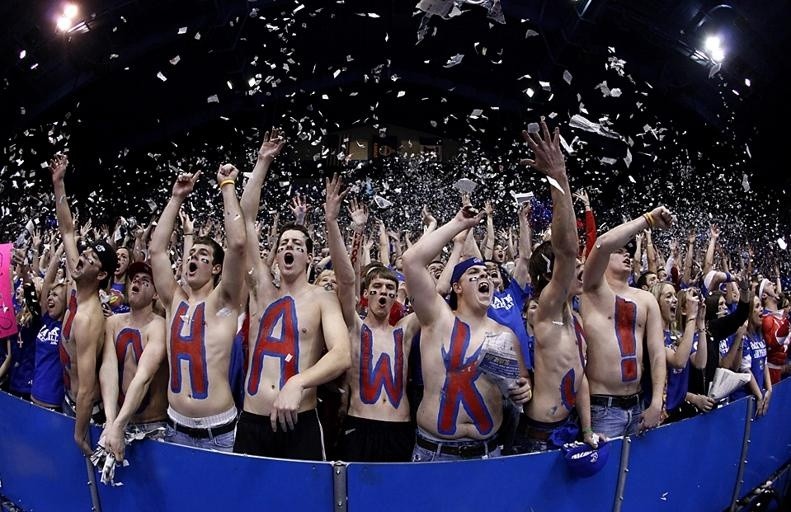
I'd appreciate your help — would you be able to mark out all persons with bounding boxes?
[11,228,44,315]
[401,204,533,462]
[673,221,725,286]
[283,194,369,305]
[356,261,405,327]
[364,237,409,316]
[657,269,669,281]
[674,287,715,418]
[482,201,506,266]
[578,207,673,439]
[389,229,414,255]
[324,171,470,462]
[133,229,148,262]
[375,217,407,281]
[177,209,196,293]
[0,336,12,390]
[731,293,772,416]
[101,245,130,316]
[47,153,118,457]
[7,248,43,401]
[755,265,782,294]
[39,229,66,281]
[518,122,604,452]
[271,211,279,235]
[725,224,753,273]
[30,234,81,413]
[461,195,533,436]
[232,127,352,461]
[645,281,708,425]
[627,257,634,285]
[574,189,596,261]
[149,163,247,455]
[97,260,166,466]
[710,292,750,408]
[419,204,445,285]
[777,293,791,315]
[72,212,110,244]
[634,227,658,285]
[637,271,659,291]
[755,277,791,383]
[705,274,750,412]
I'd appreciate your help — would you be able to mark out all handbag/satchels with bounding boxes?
[687,318,696,322]
[643,212,656,228]
[698,332,707,336]
[697,327,707,332]
[184,234,194,236]
[726,273,731,282]
[582,428,592,434]
[219,179,235,189]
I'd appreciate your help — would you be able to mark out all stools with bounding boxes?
[166,414,237,439]
[412,433,503,456]
[588,395,641,410]
